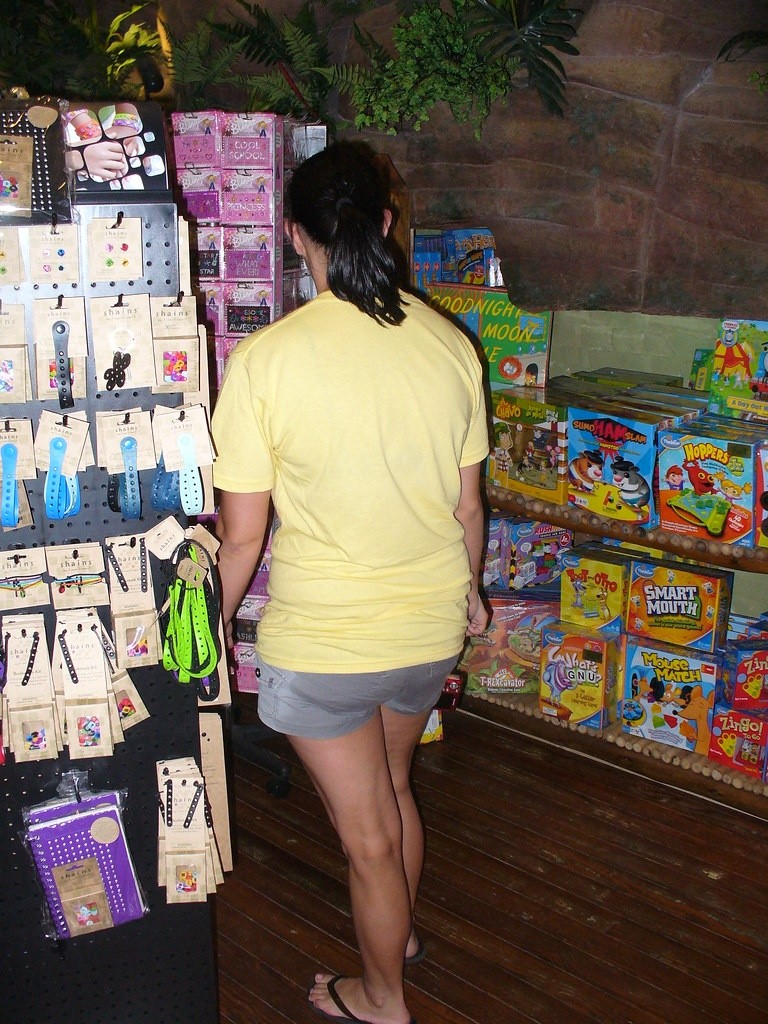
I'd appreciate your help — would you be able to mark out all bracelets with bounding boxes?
[76,119,101,140]
[114,114,138,121]
[65,108,88,122]
[113,119,139,131]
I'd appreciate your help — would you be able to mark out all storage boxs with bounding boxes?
[492,317,768,548]
[172,111,327,393]
[416,516,768,783]
[198,490,281,697]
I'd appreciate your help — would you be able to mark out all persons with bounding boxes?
[62,103,151,189]
[210,140,490,1024]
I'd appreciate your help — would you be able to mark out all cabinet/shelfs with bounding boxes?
[456,484,768,821]
